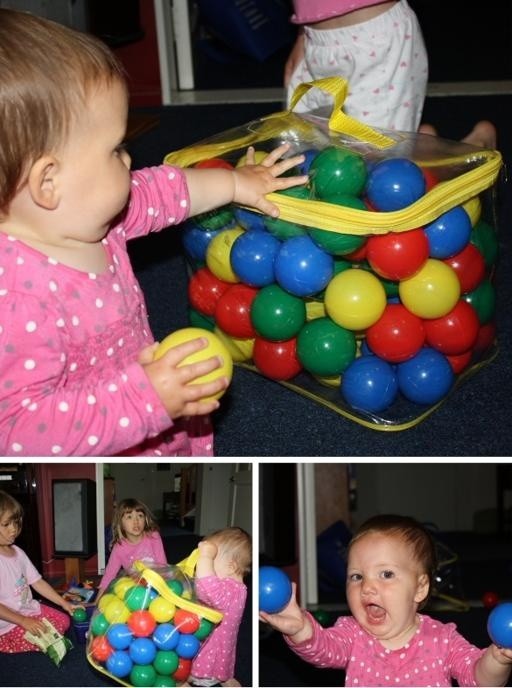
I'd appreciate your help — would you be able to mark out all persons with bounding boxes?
[80,497,171,608]
[0,490,86,654]
[284,0,497,160]
[1,10,308,458]
[185,147,498,413]
[186,527,252,686]
[259,514,512,687]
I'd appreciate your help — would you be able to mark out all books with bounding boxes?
[91,577,214,687]
[74,609,87,622]
[185,148,498,412]
[154,327,233,404]
[484,592,498,609]
[488,602,512,648]
[258,565,292,613]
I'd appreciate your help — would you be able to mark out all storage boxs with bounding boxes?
[86,546,224,686]
[163,78,505,436]
